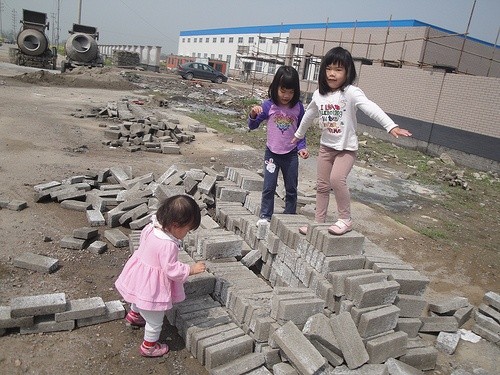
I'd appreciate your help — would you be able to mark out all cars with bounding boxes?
[177,62,228,84]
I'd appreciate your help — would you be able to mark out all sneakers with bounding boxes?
[138,341,169,358]
[124,310,146,326]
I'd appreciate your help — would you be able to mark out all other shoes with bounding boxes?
[328,219,353,235]
[256,218,267,227]
[299,226,308,235]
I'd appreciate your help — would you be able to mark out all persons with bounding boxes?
[247,65,309,228]
[115,195,206,357]
[291,47,413,236]
